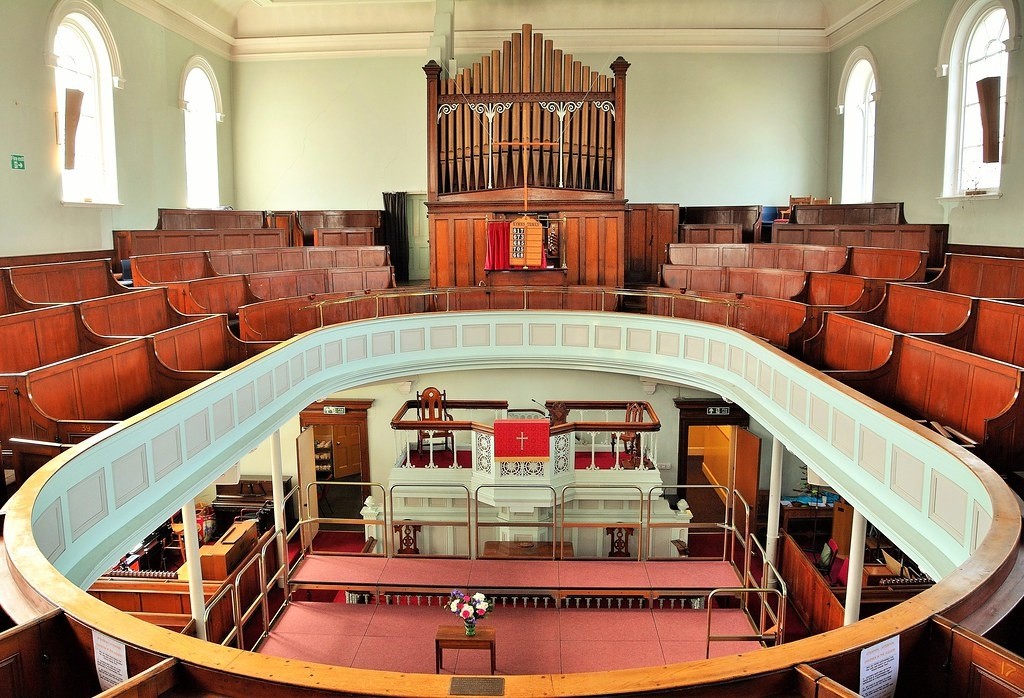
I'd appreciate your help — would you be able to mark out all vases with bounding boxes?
[464,619,476,636]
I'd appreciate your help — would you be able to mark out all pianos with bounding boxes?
[211,475,294,538]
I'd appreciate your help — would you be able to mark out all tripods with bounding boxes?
[802,486,823,563]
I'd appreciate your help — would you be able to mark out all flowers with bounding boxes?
[443,588,489,622]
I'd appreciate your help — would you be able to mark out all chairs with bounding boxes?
[610,402,643,462]
[416,386,455,458]
[605,521,634,558]
[394,519,422,554]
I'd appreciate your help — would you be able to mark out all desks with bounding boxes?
[781,495,834,549]
[435,625,497,675]
[483,541,573,559]
